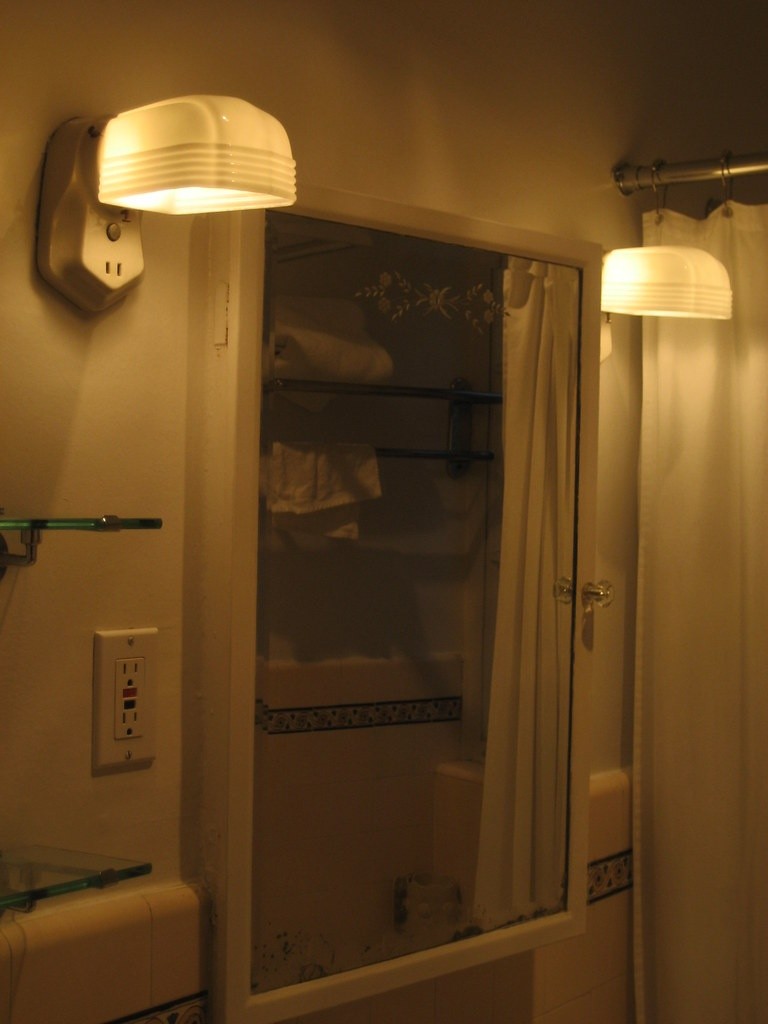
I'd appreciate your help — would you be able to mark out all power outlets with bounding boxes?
[92,628,159,770]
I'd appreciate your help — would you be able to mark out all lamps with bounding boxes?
[599,241,735,320]
[37,94,297,316]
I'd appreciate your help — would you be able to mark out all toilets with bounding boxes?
[434,760,484,884]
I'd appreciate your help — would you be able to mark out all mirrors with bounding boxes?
[209,186,590,1024]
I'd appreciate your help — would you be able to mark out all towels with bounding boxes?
[269,296,392,413]
[267,442,381,547]
